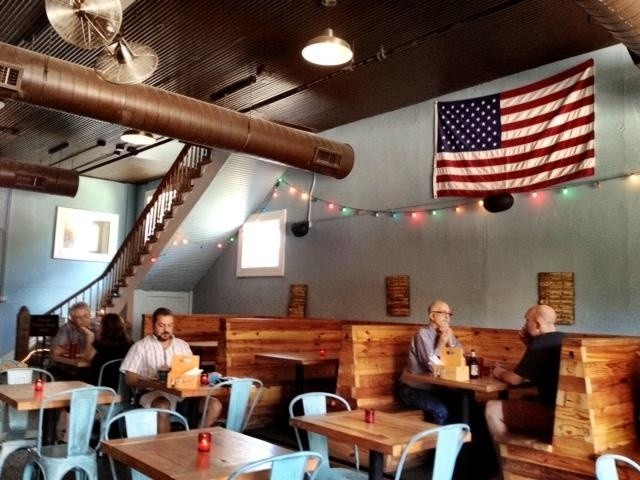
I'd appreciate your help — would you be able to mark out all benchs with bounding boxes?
[215,317,342,429]
[493,337,640,479]
[141,313,242,371]
[327,324,473,474]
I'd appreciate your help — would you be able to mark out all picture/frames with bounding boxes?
[236,209,287,278]
[51,206,120,262]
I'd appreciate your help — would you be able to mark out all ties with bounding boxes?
[434,328,442,349]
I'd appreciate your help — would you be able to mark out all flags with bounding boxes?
[432,57,597,201]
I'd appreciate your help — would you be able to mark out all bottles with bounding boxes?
[469,349,479,379]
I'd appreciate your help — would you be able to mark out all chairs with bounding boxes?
[288,391,369,480]
[595,454,640,480]
[395,423,471,480]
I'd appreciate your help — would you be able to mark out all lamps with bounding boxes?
[301,0,354,66]
[120,129,156,147]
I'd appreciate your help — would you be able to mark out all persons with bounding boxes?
[83,313,134,392]
[485,304,564,480]
[50,302,101,357]
[118,307,223,434]
[398,300,467,425]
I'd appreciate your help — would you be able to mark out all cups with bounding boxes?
[320,348,326,357]
[198,433,211,452]
[35,378,43,392]
[366,408,375,422]
[201,373,208,386]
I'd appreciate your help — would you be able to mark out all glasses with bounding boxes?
[433,311,453,317]
